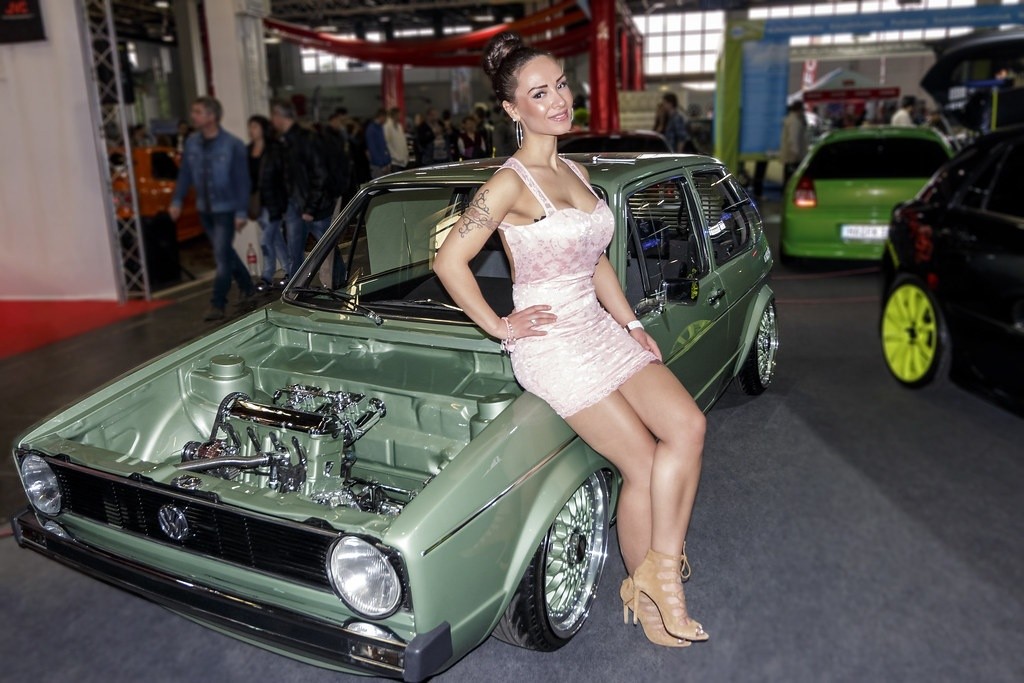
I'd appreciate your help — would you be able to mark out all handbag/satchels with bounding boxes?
[232,220,282,282]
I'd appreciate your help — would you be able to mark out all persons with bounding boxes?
[170,97,257,320]
[172,119,195,149]
[652,91,707,157]
[431,31,709,648]
[288,94,521,235]
[244,115,290,293]
[120,123,157,148]
[779,93,941,193]
[273,101,348,293]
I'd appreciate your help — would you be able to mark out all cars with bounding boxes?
[11,152,780,683]
[108,147,205,243]
[879,128,1024,420]
[780,124,958,272]
[556,131,672,157]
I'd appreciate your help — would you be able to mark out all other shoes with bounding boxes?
[231,285,255,306]
[254,280,277,297]
[203,304,224,319]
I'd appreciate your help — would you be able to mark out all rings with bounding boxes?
[530,320,536,326]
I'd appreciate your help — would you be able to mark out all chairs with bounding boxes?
[626,219,669,308]
[400,205,514,318]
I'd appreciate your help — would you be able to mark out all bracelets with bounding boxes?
[501,316,516,356]
[623,320,644,333]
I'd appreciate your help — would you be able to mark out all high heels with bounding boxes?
[619,575,691,647]
[633,541,709,640]
